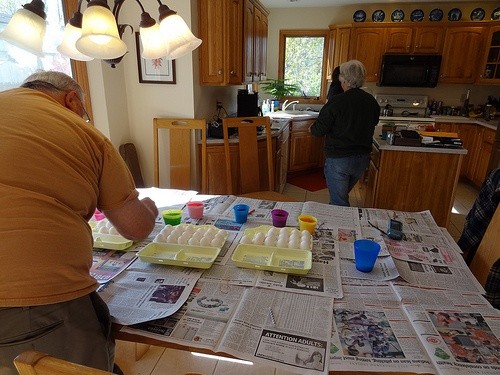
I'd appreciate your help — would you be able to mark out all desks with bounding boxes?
[88,186,500,375]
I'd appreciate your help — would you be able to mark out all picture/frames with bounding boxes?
[134,32,176,84]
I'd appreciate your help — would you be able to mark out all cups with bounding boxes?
[298,214,318,235]
[270,209,289,227]
[93,210,104,220]
[484,70,492,78]
[233,204,249,224]
[354,239,381,272]
[162,209,182,226]
[186,201,204,218]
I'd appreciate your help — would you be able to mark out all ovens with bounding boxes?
[377,118,435,130]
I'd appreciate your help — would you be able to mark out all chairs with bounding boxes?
[223,117,293,204]
[152,118,208,191]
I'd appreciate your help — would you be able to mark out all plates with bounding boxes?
[409,8,425,22]
[391,8,404,22]
[447,7,462,21]
[372,9,385,22]
[429,8,443,21]
[352,9,366,22]
[490,7,500,20]
[470,7,485,21]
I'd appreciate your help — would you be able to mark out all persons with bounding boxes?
[308,60,380,207]
[0,71,159,375]
[306,351,323,370]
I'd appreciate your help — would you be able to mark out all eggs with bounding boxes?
[95,220,120,235]
[240,227,311,250]
[155,224,227,247]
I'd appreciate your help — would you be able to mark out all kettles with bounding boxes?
[382,104,393,114]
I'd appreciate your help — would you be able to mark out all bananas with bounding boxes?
[468,111,483,117]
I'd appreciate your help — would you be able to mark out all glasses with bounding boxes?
[76,95,91,123]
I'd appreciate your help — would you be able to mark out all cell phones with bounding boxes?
[387,219,403,240]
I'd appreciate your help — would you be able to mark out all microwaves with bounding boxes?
[378,52,442,86]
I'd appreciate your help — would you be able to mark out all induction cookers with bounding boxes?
[373,93,435,122]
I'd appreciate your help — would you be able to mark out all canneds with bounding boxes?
[432,101,452,115]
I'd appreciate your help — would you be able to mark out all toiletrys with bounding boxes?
[263,104,270,117]
[262,100,269,113]
[271,101,274,112]
[266,99,270,112]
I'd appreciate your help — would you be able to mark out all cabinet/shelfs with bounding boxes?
[473,124,495,185]
[290,120,321,171]
[244,0,261,82]
[262,4,269,80]
[365,144,380,208]
[440,27,490,83]
[199,0,244,86]
[450,125,475,177]
[276,124,290,193]
[326,28,350,79]
[478,26,500,84]
[351,28,385,83]
[386,27,442,53]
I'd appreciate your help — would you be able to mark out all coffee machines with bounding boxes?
[236,89,263,133]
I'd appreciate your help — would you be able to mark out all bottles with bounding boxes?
[425,89,500,121]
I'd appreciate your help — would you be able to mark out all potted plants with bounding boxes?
[260,79,297,110]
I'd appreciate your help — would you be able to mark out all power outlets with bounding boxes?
[216,100,218,111]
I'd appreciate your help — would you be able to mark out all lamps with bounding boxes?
[0,0,202,69]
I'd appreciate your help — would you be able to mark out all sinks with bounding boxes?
[282,110,319,117]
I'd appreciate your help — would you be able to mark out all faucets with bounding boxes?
[292,104,296,111]
[281,99,299,112]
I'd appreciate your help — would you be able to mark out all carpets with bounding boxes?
[288,169,328,192]
